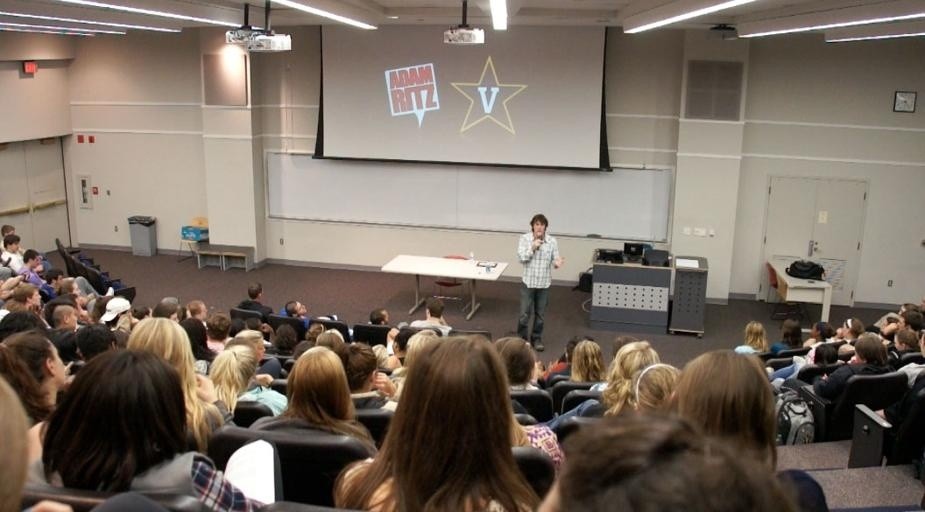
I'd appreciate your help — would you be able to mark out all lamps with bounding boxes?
[489,0,508,30]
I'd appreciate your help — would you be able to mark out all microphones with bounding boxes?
[536,232,541,250]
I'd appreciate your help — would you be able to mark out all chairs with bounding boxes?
[177,217,209,263]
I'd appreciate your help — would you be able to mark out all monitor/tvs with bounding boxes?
[624,243,643,261]
[645,250,668,266]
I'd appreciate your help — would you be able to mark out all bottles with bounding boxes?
[775,434,783,446]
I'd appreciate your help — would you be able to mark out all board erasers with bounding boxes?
[587,234,602,238]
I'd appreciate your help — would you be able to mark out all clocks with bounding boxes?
[893,90,917,113]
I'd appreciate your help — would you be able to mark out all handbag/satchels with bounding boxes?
[775,392,815,446]
[578,267,593,292]
[786,260,826,281]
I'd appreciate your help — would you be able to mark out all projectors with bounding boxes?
[444,29,485,44]
[247,34,292,51]
[226,30,276,44]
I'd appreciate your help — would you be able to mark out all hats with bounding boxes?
[100,297,131,321]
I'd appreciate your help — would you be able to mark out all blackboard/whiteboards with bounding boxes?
[267,151,674,243]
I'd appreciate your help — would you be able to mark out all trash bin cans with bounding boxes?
[128,215,156,256]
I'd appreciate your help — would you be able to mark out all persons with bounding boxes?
[0,215,925,512]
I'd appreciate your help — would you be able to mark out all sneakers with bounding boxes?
[105,287,114,297]
[533,339,544,351]
